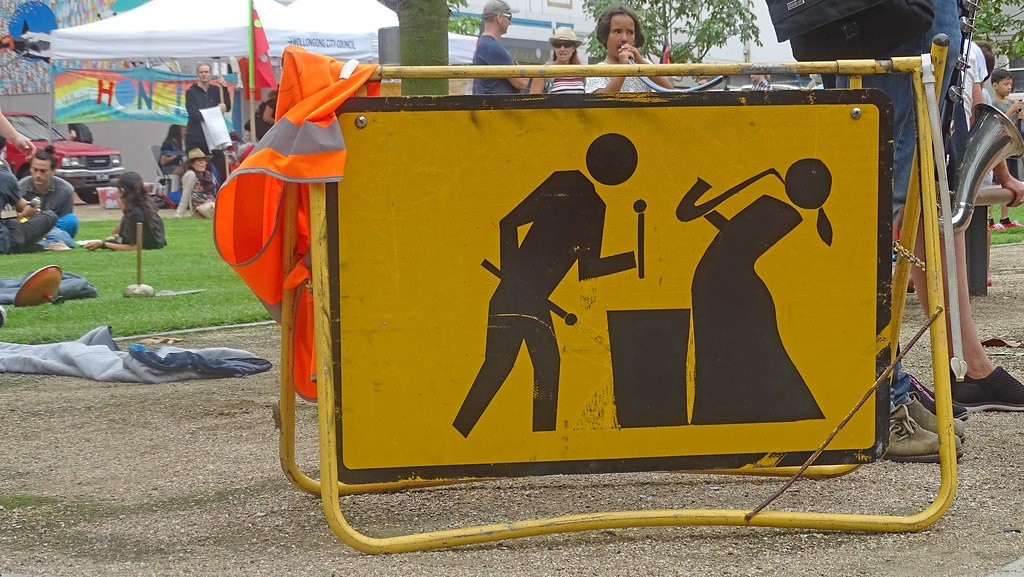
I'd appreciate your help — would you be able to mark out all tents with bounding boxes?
[48,1,403,162]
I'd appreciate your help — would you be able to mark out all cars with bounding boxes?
[0,111,125,205]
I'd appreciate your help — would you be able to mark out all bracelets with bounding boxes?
[103,241,105,247]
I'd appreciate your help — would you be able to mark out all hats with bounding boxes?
[549,27,582,48]
[111,172,143,189]
[483,0,520,18]
[183,148,213,170]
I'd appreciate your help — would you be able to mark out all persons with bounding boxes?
[0,63,279,251]
[809,75,823,90]
[697,75,713,89]
[585,5,673,94]
[767,0,1024,462]
[471,0,528,94]
[750,75,771,91]
[529,26,585,95]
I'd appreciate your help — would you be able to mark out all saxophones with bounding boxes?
[931,0,1024,233]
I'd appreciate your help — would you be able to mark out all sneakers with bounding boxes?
[907,374,969,420]
[881,404,963,462]
[988,223,1004,230]
[894,390,966,442]
[953,366,1024,411]
[1001,220,1024,228]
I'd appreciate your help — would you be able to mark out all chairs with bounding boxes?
[149,144,172,195]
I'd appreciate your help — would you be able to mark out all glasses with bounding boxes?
[553,42,576,48]
[501,14,512,21]
